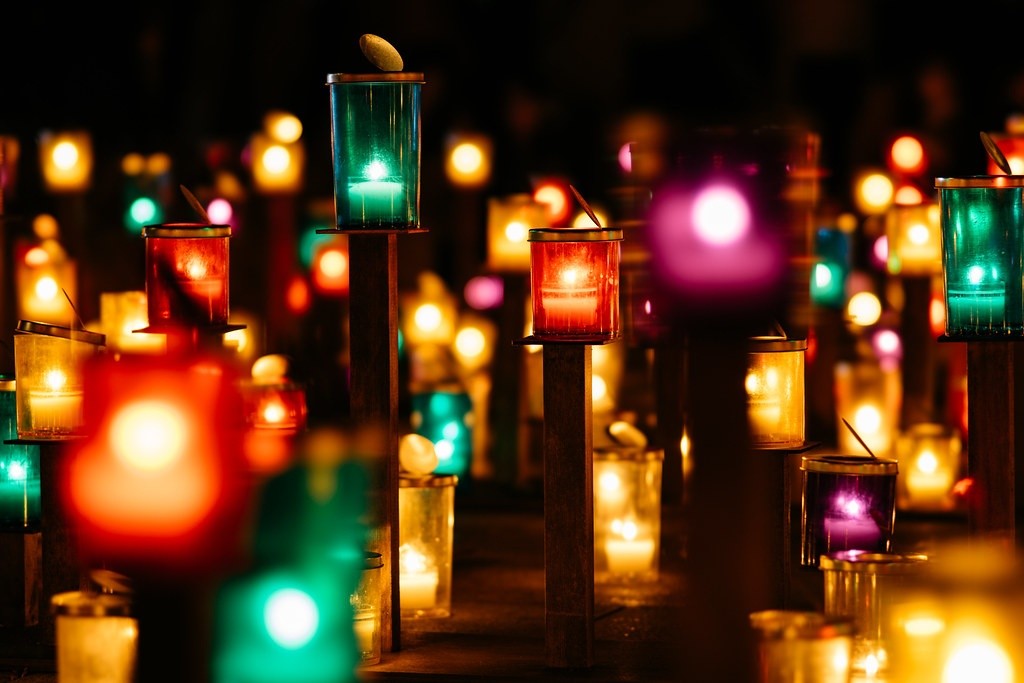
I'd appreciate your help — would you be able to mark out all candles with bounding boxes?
[349,592,375,652]
[825,499,880,546]
[542,266,596,323]
[900,224,935,271]
[605,520,654,570]
[947,265,1005,322]
[30,369,83,433]
[399,543,438,608]
[748,369,786,430]
[169,259,221,319]
[25,276,71,323]
[0,461,40,513]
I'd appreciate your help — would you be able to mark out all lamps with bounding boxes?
[346,160,405,223]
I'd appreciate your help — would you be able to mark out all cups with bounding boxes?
[875,563,1024,683]
[13,335,98,439]
[751,611,862,683]
[326,74,424,228]
[746,337,805,450]
[938,180,1024,340]
[143,223,232,327]
[57,618,138,683]
[820,553,932,639]
[398,474,456,620]
[799,455,899,568]
[593,451,665,587]
[528,227,623,341]
[0,377,40,532]
[352,552,386,666]
